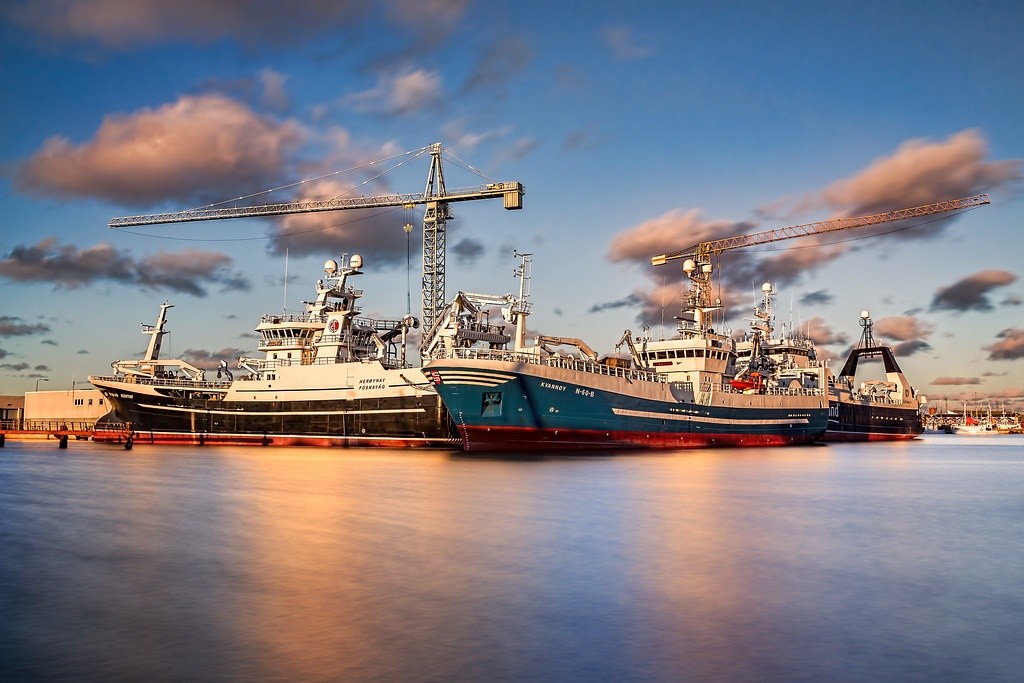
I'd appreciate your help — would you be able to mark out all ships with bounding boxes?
[421,248,830,457]
[88,253,462,441]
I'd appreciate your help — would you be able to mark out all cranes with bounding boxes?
[107,142,527,367]
[651,192,992,337]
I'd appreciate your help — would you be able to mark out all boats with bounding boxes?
[729,371,766,391]
[815,311,927,442]
[921,391,1024,436]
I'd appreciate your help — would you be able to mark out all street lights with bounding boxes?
[36,378,49,392]
